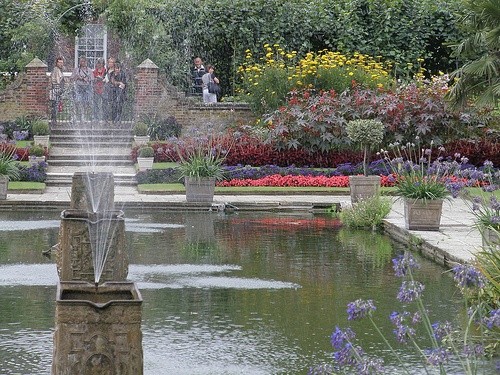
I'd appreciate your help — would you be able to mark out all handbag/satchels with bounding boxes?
[207,74,219,93]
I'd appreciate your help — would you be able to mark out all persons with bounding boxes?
[70,55,128,122]
[201,63,219,103]
[51,57,65,122]
[190,57,205,97]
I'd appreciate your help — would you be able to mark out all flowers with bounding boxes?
[377,135,458,202]
[158,127,237,176]
[457,160,500,236]
[0,131,31,179]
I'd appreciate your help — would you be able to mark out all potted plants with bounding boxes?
[345,118,383,202]
[34,121,50,147]
[133,122,151,144]
[137,147,154,170]
[27,147,46,165]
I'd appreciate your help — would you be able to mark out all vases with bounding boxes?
[480,229,500,254]
[403,197,442,230]
[0,177,9,200]
[186,177,215,202]
